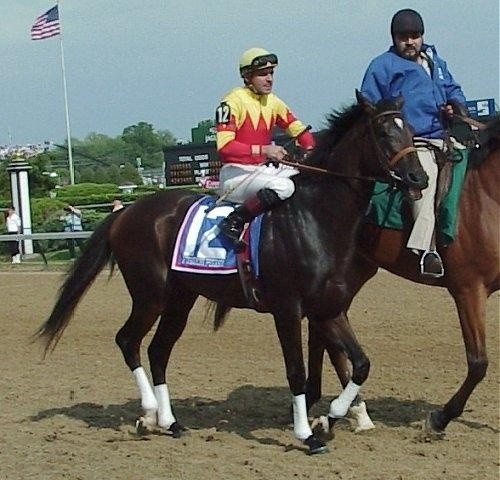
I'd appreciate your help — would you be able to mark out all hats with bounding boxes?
[390,8,425,35]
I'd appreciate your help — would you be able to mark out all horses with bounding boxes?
[202,110,500,440]
[28,89,430,455]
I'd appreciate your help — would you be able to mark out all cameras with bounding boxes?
[63,208,71,211]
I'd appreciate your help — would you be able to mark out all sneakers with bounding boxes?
[419,254,441,285]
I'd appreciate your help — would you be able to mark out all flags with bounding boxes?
[29,6,59,40]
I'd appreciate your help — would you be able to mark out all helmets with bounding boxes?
[239,46,279,76]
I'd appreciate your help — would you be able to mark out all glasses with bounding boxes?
[252,54,278,67]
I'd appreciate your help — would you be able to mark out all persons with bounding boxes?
[361,8,468,285]
[6,207,21,263]
[113,198,125,212]
[214,46,314,245]
[59,203,86,258]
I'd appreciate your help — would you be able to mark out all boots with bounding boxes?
[217,187,283,250]
